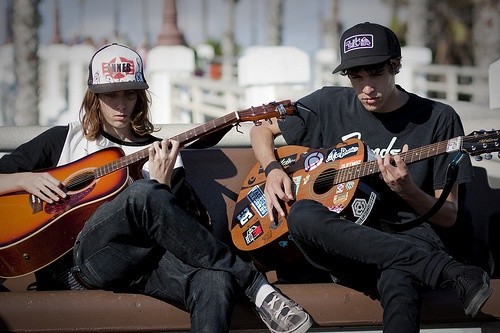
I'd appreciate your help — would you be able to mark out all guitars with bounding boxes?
[0,100,301,279]
[230,128,500,258]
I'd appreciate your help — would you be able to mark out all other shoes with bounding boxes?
[451,265,492,320]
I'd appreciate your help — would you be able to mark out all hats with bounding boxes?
[333,22,400,73]
[88,43,149,93]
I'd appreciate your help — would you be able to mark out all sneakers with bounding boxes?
[256,290,312,333]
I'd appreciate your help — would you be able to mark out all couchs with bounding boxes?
[0,123,500,333]
